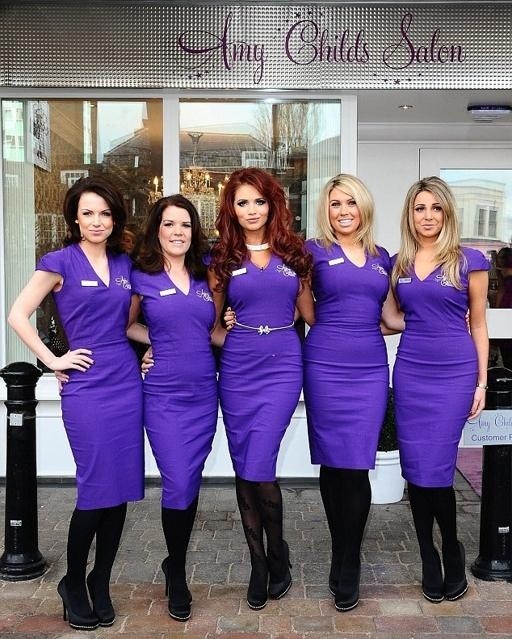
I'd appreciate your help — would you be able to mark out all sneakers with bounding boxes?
[329,550,360,610]
[421,542,468,602]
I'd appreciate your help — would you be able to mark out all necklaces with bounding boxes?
[243,241,269,253]
[253,262,268,274]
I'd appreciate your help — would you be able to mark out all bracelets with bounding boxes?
[477,382,490,393]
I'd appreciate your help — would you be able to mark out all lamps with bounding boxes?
[467,101,511,123]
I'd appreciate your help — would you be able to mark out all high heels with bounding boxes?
[57,572,114,629]
[247,541,292,610]
[160,555,192,620]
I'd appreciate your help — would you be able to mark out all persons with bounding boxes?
[120,229,138,252]
[210,167,313,610]
[6,174,144,630]
[486,246,512,374]
[380,175,491,606]
[222,173,473,614]
[125,195,218,621]
